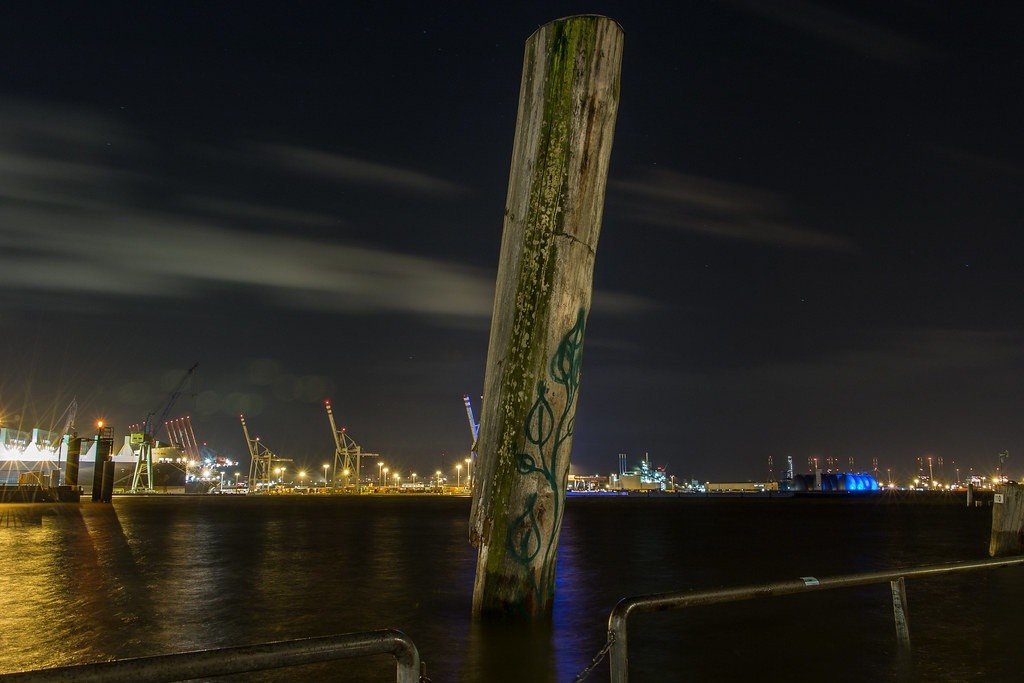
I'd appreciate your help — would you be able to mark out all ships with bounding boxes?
[1,434,188,495]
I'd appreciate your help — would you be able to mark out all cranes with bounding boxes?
[241,413,277,498]
[323,399,363,497]
[129,361,200,493]
